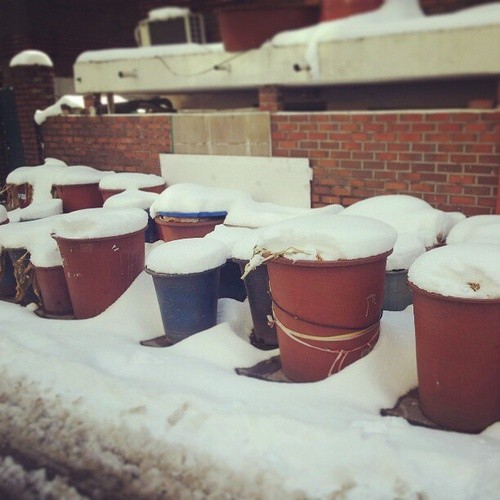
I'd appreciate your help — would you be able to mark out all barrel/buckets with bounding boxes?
[0,168,500,435]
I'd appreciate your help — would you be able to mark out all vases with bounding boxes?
[209,0,321,52]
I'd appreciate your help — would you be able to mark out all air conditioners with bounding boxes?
[137,12,207,46]
